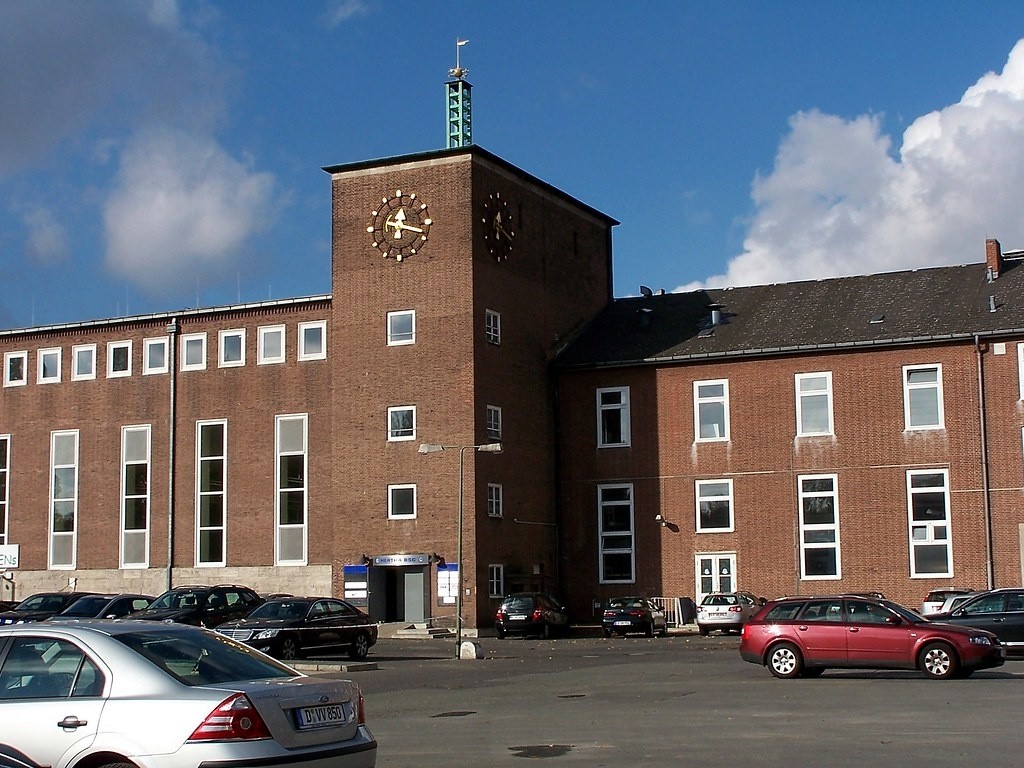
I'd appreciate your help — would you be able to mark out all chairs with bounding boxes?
[208,598,222,611]
[712,599,730,605]
[181,598,196,609]
[803,611,815,621]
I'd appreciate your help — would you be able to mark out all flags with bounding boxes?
[458,38,469,47]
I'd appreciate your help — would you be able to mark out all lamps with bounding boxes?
[428,553,439,563]
[361,554,369,565]
[655,514,667,525]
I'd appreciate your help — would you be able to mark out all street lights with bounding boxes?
[418,443,503,659]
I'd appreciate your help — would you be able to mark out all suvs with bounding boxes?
[120,583,269,630]
[696,590,768,635]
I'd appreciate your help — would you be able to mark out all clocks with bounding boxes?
[365,191,432,261]
[482,191,515,263]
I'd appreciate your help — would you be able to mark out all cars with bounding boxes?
[0,619,378,768]
[919,586,1024,663]
[602,597,669,638]
[213,592,379,660]
[495,592,571,640]
[739,592,1006,679]
[0,592,167,626]
